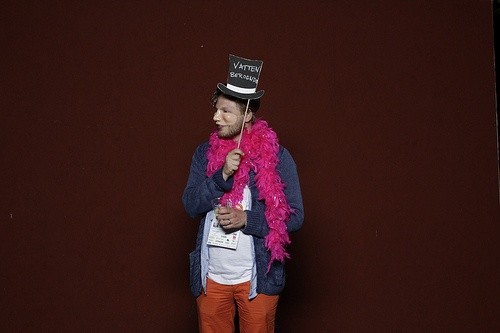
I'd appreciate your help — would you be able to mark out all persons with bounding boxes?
[182,54,305,333]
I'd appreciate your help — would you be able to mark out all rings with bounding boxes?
[228,219,232,225]
